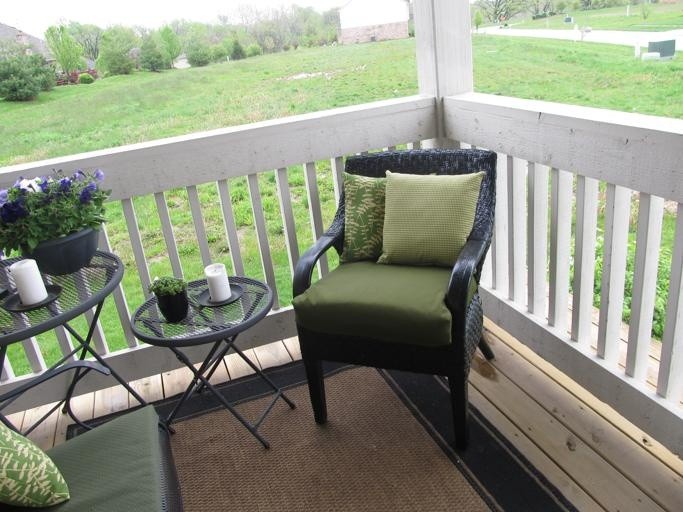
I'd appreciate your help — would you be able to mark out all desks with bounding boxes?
[0,249,175,434]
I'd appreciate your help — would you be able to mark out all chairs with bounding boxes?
[0,360,182,512]
[292,149,497,448]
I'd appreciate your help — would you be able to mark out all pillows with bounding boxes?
[376,170,486,276]
[0,418,69,507]
[339,172,436,264]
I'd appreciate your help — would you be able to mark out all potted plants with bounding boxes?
[148,277,188,323]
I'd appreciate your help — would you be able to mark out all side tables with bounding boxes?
[130,276,295,449]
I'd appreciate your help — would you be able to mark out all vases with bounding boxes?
[20,227,98,275]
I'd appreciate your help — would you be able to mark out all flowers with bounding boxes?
[0,167,111,257]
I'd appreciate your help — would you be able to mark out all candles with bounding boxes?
[204,263,231,302]
[9,259,48,306]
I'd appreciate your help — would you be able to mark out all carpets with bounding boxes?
[64,359,577,511]
